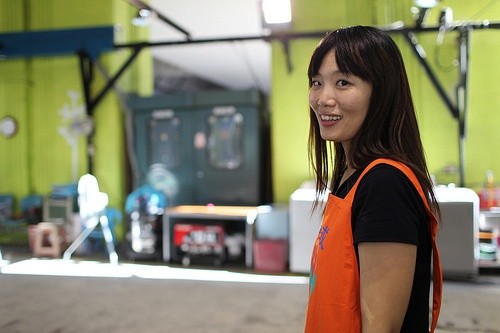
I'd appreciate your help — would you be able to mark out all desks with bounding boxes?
[159,204,271,266]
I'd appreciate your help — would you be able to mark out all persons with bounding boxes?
[303,25,443,332]
